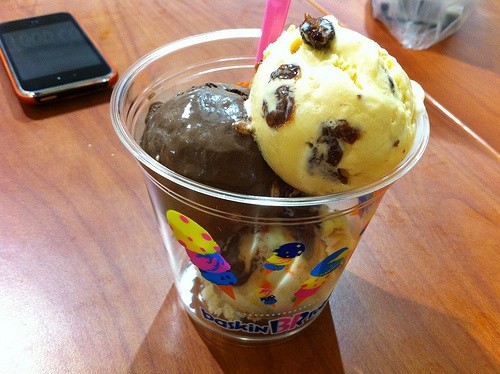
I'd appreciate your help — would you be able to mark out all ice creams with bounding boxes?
[139,14,418,323]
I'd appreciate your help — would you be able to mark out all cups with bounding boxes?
[109,28,431,351]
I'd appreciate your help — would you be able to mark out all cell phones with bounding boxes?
[1,12,118,104]
